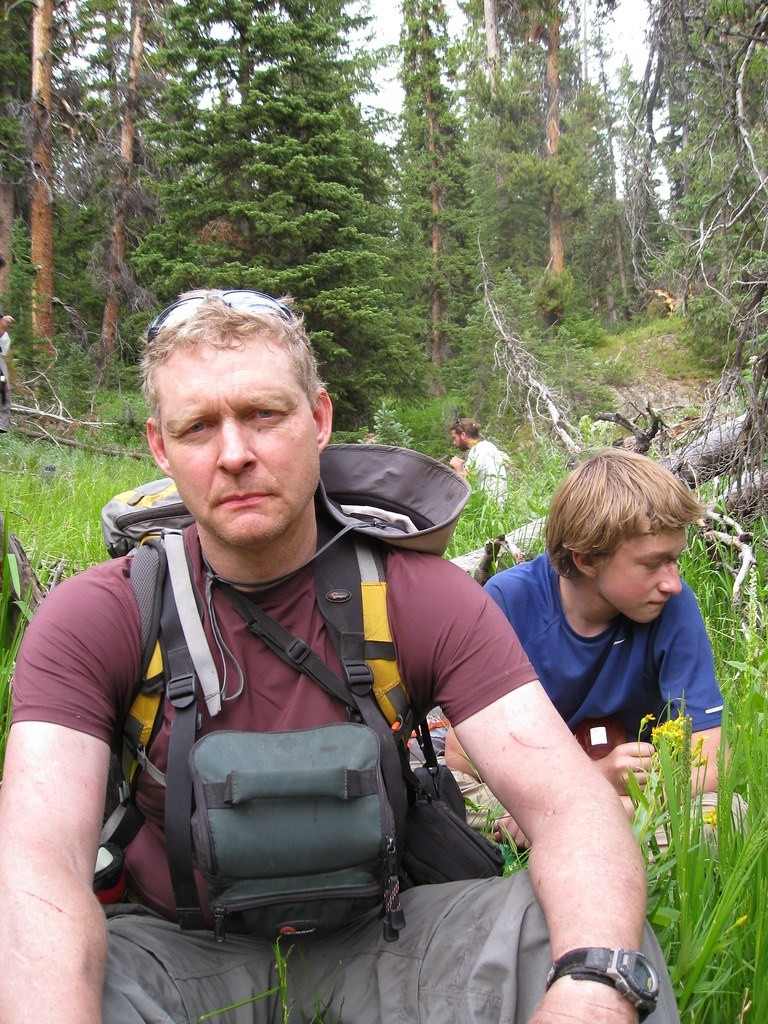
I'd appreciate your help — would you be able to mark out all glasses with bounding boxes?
[146,290,294,347]
[456,418,464,431]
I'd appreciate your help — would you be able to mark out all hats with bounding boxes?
[318,444,472,557]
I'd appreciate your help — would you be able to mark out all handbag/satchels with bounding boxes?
[397,763,503,889]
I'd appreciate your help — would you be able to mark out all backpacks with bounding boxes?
[101,476,410,845]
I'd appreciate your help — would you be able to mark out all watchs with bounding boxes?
[546,947,661,1024]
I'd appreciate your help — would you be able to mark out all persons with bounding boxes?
[449,418,508,512]
[0,252,13,432]
[411,451,730,846]
[0,289,680,1024]
